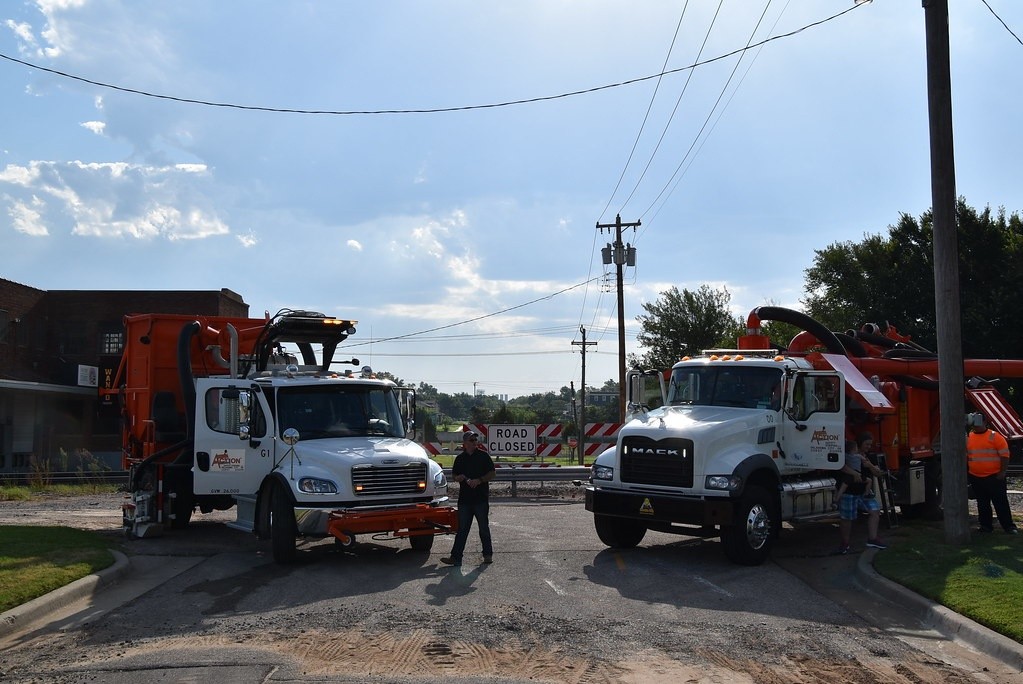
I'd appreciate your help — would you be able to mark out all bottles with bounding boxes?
[462,475,477,488]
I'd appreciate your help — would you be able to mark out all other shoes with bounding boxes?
[1007,526,1018,535]
[440,555,463,566]
[981,526,993,532]
[484,553,493,562]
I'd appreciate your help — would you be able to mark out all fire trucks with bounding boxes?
[570,303,1023,567]
[96,307,461,567]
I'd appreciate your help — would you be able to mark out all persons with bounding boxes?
[832,432,888,553]
[440,431,496,566]
[966,411,1018,534]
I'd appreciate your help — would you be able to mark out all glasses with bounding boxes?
[466,438,477,443]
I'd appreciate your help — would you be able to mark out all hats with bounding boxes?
[463,431,478,442]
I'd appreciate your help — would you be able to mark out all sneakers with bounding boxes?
[840,542,850,554]
[866,538,887,549]
